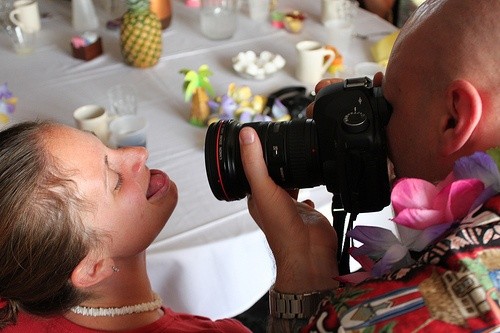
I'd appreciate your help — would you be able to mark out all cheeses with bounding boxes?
[232,50,286,80]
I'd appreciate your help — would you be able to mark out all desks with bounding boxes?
[0,0,412,320]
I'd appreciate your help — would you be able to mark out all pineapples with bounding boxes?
[119,0,162,67]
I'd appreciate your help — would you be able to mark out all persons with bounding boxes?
[362,0,425,29]
[0,121,256,332]
[239,0,500,333]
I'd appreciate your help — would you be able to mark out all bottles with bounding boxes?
[151,0,176,31]
[71,0,99,32]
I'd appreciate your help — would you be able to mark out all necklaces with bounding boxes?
[71,293,161,316]
[331,145,499,288]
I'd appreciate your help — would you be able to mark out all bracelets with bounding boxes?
[269,285,323,319]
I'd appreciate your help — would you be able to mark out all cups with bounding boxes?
[199,0,240,40]
[108,115,150,147]
[107,83,138,116]
[73,104,109,145]
[296,40,336,83]
[9,0,41,34]
[321,0,350,28]
[4,23,35,55]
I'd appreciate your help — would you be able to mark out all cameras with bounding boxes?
[203,78,393,216]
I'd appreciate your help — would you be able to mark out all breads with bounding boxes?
[369,31,399,65]
